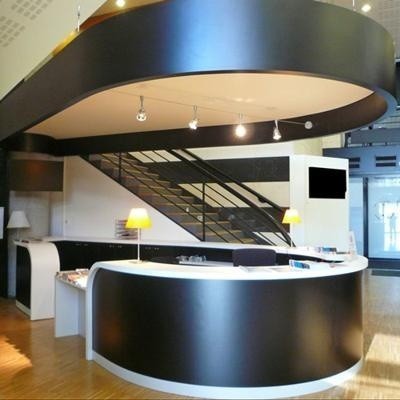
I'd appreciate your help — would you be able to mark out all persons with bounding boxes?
[386,212,398,236]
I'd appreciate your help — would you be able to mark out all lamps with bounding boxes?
[6,209,32,242]
[282,208,302,248]
[272,120,282,141]
[136,96,151,122]
[125,207,152,264]
